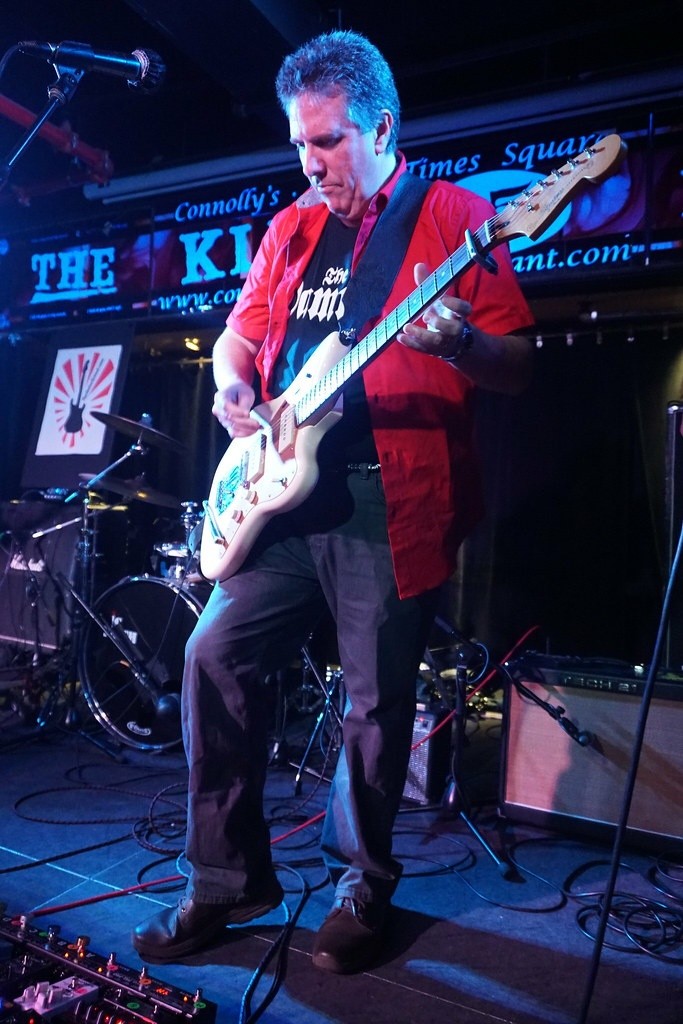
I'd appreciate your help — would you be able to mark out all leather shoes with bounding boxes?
[130,891,282,960]
[313,898,390,975]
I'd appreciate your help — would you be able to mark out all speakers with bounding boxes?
[494,659,683,854]
[402,698,503,807]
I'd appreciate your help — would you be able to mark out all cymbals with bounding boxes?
[90,408,184,452]
[79,471,188,511]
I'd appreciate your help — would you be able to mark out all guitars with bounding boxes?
[199,134,626,579]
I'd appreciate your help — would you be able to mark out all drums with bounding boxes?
[79,578,200,752]
[149,539,205,591]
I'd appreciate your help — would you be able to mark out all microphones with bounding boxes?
[18,39,167,93]
[6,527,44,534]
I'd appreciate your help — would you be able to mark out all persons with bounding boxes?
[133,32,537,972]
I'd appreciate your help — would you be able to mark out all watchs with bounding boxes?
[439,320,473,361]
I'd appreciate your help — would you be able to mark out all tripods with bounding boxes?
[0,441,147,754]
